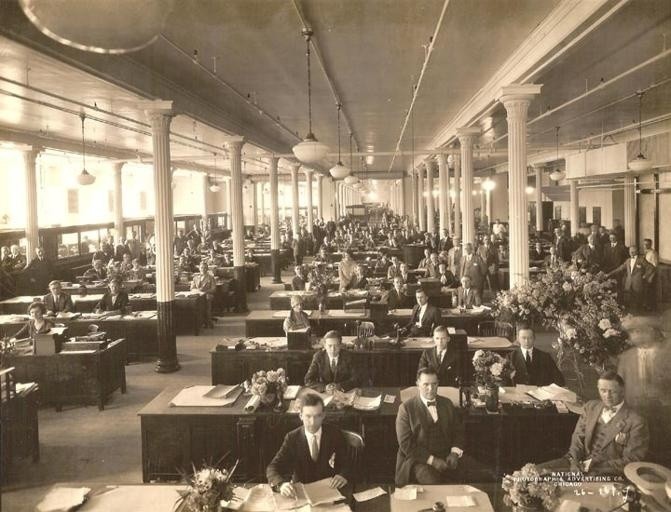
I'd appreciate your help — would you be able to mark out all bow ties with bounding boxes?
[427,402,435,406]
[110,293,116,297]
[604,406,616,412]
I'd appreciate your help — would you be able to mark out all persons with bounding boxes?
[22,247,57,294]
[452,204,509,310]
[9,244,27,271]
[174,225,217,313]
[303,330,363,392]
[0,246,12,268]
[396,287,444,339]
[42,280,73,313]
[281,294,313,337]
[394,365,472,488]
[83,231,155,281]
[415,326,475,386]
[505,326,567,388]
[265,393,351,498]
[292,215,452,259]
[94,280,129,316]
[527,216,661,315]
[410,259,452,287]
[217,215,292,276]
[292,259,368,293]
[12,302,54,342]
[373,259,410,307]
[531,371,651,482]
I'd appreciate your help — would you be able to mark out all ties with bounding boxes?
[464,289,467,304]
[312,435,319,463]
[331,358,336,377]
[55,296,58,313]
[415,306,421,323]
[526,350,531,374]
[438,351,442,365]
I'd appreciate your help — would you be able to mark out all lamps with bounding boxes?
[78,113,95,186]
[209,151,286,195]
[289,25,330,165]
[627,86,655,175]
[550,125,565,183]
[328,102,371,196]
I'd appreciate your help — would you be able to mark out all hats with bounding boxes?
[624,461,671,495]
[291,295,302,308]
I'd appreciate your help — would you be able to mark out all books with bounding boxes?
[273,477,347,512]
[202,384,240,398]
[15,382,40,398]
[526,381,577,404]
[335,390,382,411]
